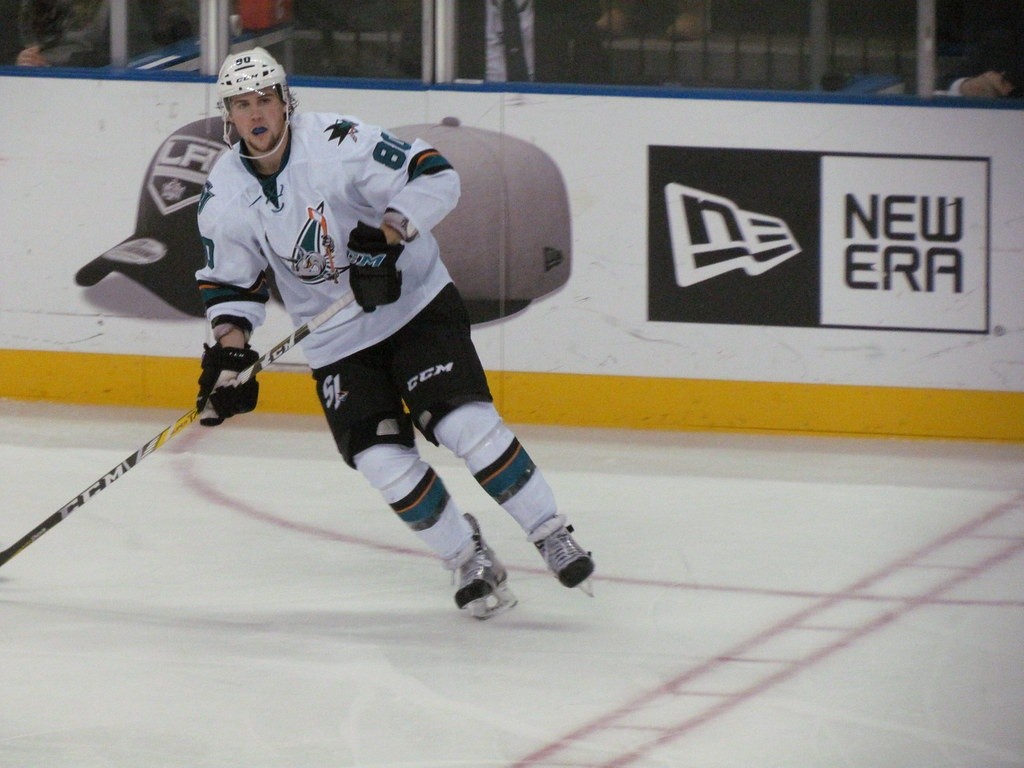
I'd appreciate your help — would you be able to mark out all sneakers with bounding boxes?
[525,513,597,598]
[441,513,519,621]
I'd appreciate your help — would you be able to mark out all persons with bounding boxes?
[194,46,597,622]
[1,0,1024,110]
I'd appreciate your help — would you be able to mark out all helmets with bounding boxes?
[216,47,299,159]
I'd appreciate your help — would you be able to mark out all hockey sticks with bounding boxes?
[0,288,356,569]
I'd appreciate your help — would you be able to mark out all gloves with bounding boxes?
[196,342,260,426]
[346,220,404,313]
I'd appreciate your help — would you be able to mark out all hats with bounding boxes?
[73,115,242,318]
[382,115,572,325]
